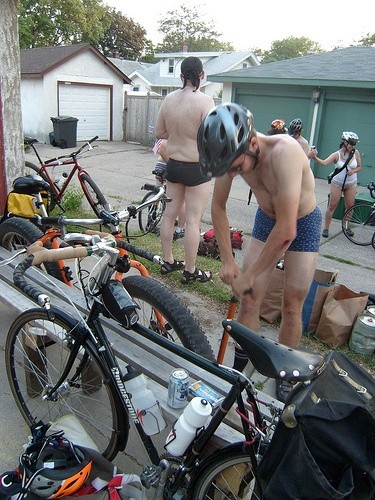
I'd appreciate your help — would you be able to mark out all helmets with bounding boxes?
[289,119,303,132]
[15,429,92,500]
[197,102,249,179]
[271,119,286,131]
[341,131,359,142]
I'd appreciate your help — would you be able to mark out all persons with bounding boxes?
[155,55,216,285]
[270,118,318,160]
[196,103,323,404]
[311,132,362,238]
[146,138,170,232]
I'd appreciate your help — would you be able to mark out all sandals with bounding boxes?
[160,259,186,275]
[180,268,212,284]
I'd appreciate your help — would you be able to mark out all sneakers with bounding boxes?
[275,378,293,403]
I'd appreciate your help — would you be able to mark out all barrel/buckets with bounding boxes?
[348,306,375,356]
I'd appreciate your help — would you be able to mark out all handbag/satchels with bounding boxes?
[250,348,375,500]
[327,170,335,184]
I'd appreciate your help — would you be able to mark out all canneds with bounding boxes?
[167,368,189,410]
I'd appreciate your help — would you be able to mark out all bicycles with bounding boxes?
[341,180,375,248]
[24,135,119,234]
[0,174,215,363]
[137,166,171,233]
[5,230,327,500]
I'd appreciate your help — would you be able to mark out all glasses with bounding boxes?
[228,153,247,173]
[346,140,356,146]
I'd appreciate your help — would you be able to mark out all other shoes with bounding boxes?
[346,229,354,236]
[322,229,329,238]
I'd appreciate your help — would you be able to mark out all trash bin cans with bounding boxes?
[50,116,79,149]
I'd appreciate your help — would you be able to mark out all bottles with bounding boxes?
[165,396,213,457]
[123,364,167,436]
[57,173,69,188]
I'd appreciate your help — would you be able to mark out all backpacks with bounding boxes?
[196,226,243,261]
[0,442,148,500]
